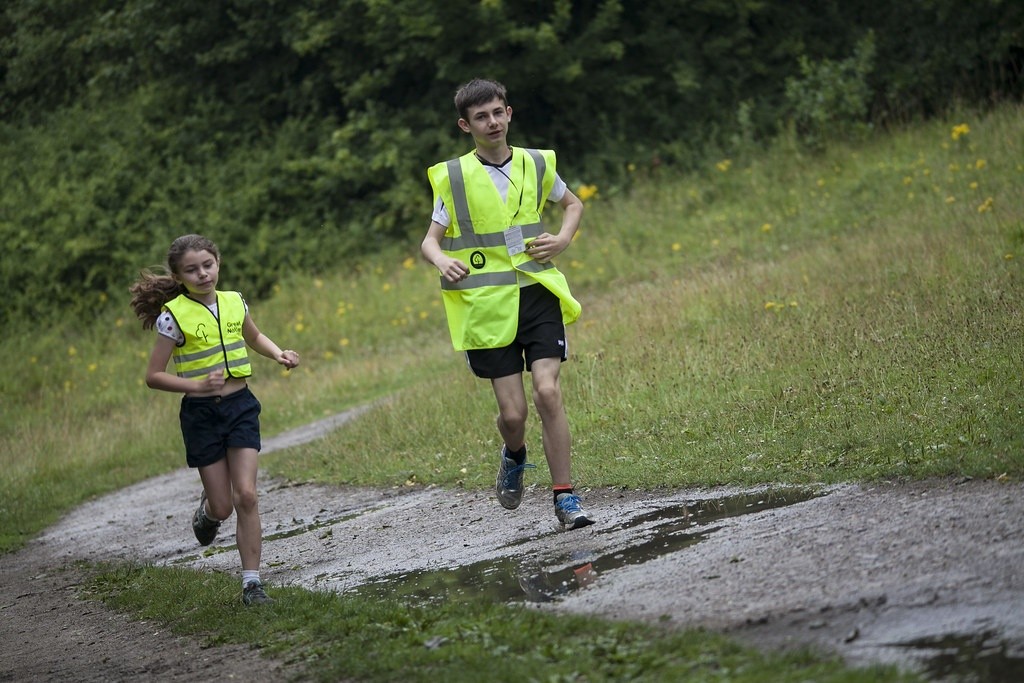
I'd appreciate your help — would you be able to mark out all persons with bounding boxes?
[129,236,299,607]
[420,78,594,529]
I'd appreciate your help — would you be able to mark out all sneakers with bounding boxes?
[191,489,222,546]
[554,493,597,531]
[496,443,537,510]
[241,579,276,608]
